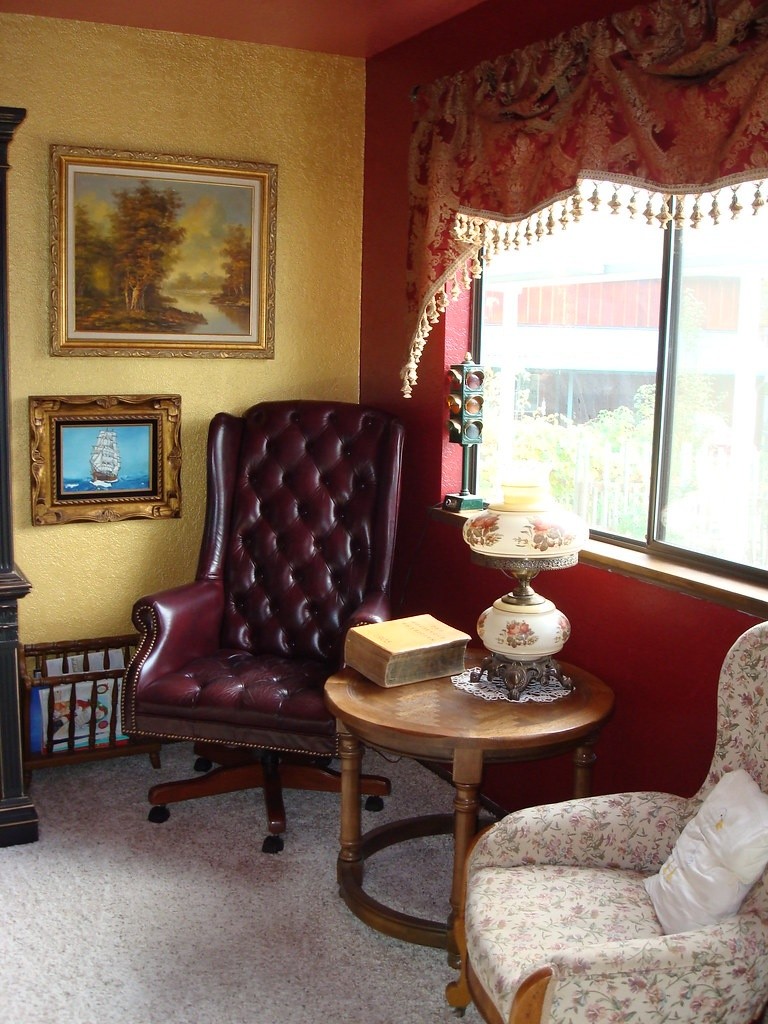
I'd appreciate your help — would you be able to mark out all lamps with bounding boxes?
[442,352,485,513]
[463,464,590,700]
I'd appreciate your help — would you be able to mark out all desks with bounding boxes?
[324,649,615,968]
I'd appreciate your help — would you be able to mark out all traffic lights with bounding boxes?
[461,363,484,445]
[447,364,464,443]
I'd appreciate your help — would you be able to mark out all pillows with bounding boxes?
[642,769,767,934]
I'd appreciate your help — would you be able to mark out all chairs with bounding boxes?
[446,622,768,1024]
[121,400,406,854]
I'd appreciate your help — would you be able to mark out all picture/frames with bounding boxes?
[49,143,278,360]
[28,394,184,526]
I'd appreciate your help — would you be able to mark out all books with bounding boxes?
[27,649,132,753]
[344,613,471,688]
